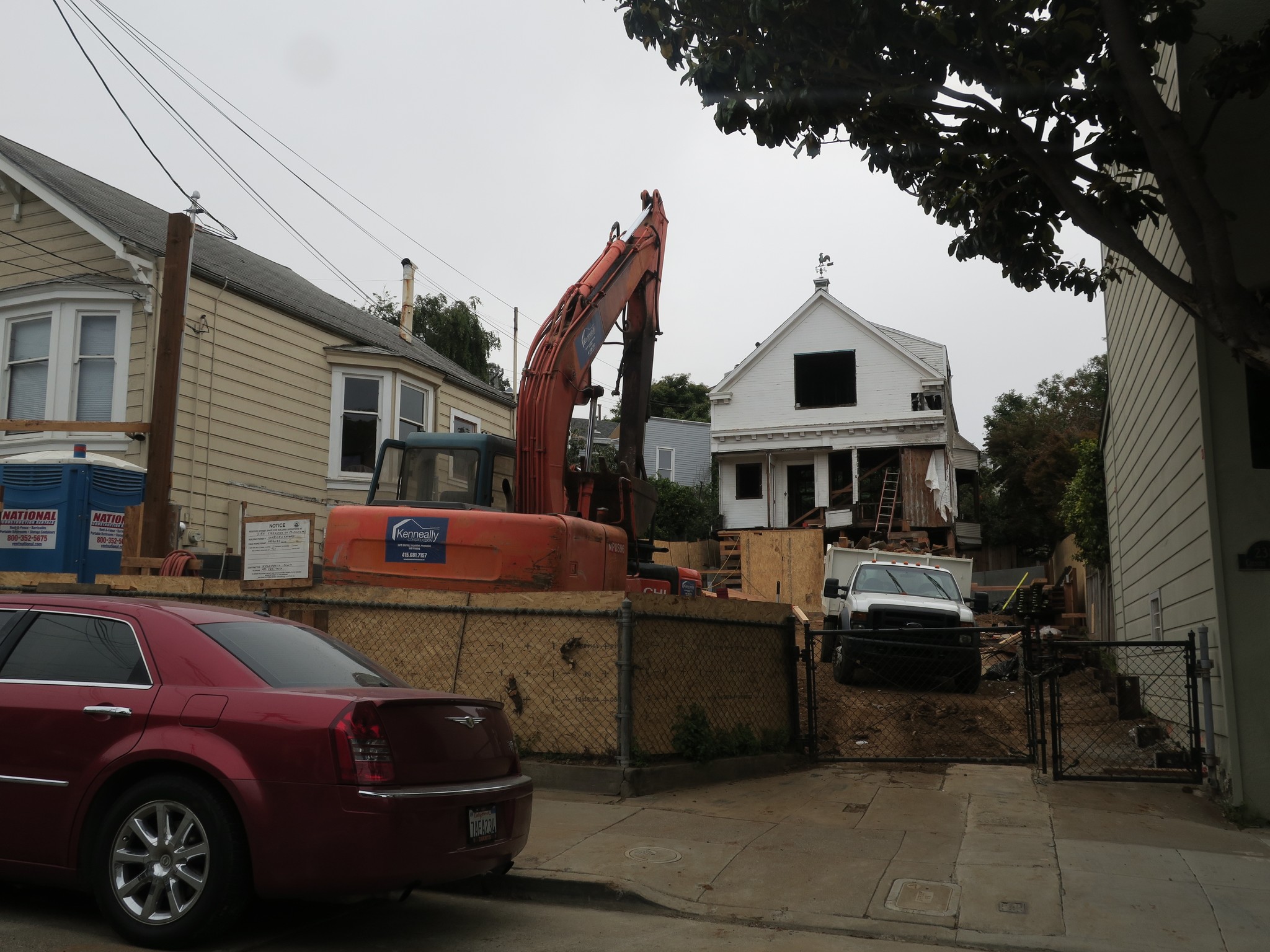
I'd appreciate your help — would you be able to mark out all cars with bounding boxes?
[0,593,535,951]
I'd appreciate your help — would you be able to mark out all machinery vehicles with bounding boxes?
[320,187,704,595]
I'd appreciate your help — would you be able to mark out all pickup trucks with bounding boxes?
[820,544,990,694]
